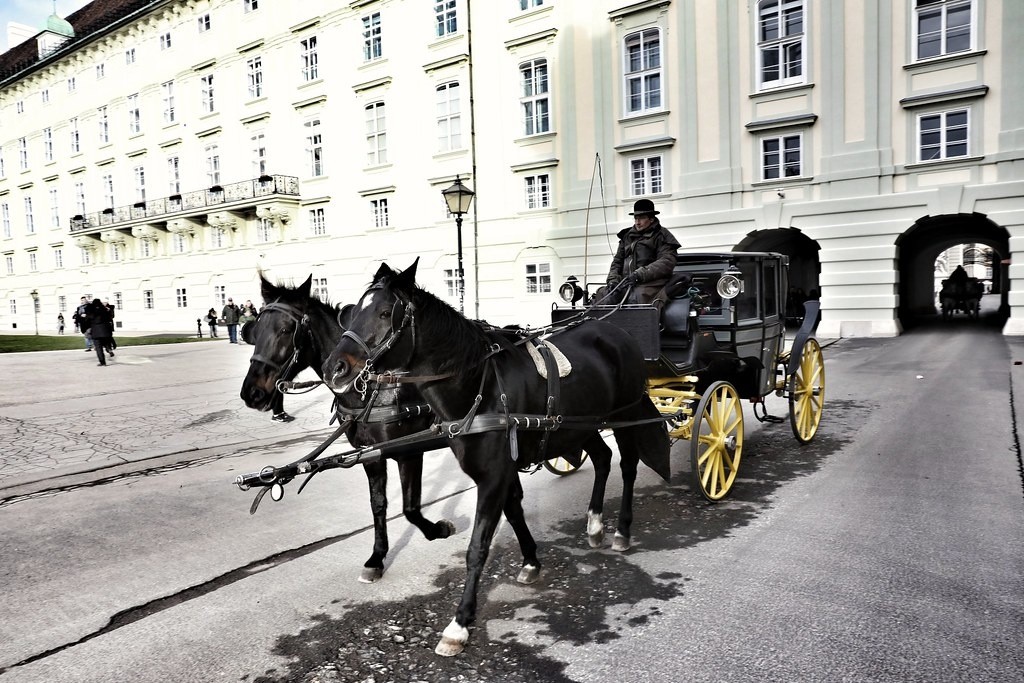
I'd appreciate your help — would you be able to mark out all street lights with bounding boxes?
[30,290,39,336]
[440,173,476,315]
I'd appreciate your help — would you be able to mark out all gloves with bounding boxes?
[605,282,618,295]
[619,274,641,289]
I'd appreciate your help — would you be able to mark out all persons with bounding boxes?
[207,308,218,338]
[596,199,681,309]
[72,297,117,366]
[271,393,295,424]
[57,313,65,335]
[949,265,967,281]
[221,297,258,344]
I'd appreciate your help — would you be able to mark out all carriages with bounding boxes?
[232,250,826,658]
[940,277,984,320]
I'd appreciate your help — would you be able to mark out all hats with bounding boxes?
[629,199,660,216]
[228,298,232,301]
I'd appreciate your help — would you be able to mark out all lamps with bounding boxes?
[559,275,582,310]
[717,265,742,326]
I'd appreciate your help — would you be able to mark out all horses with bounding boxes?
[942,282,954,322]
[240,270,525,583]
[321,255,646,656]
[963,281,978,323]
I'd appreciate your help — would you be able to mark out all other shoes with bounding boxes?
[97,362,106,366]
[113,344,116,349]
[230,342,238,343]
[211,336,213,338]
[86,349,91,351]
[110,352,114,357]
[215,336,218,337]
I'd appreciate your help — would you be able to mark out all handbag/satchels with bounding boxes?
[204,315,212,323]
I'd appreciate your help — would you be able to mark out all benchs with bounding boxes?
[595,286,691,349]
